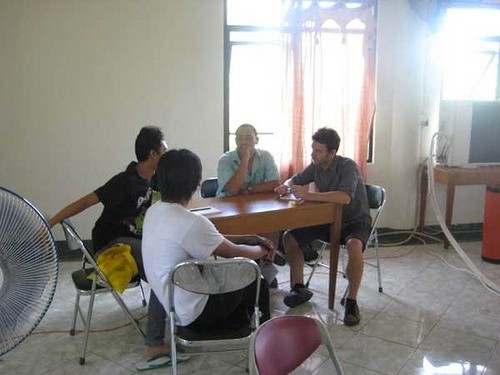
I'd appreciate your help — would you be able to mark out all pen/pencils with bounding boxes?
[190,208,211,212]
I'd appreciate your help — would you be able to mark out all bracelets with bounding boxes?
[276,188,278,195]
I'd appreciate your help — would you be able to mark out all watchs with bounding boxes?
[247,183,254,195]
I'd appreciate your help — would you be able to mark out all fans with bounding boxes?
[0,186,59,356]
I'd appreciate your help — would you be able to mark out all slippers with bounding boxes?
[136,352,191,370]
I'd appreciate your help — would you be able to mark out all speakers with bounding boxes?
[437,101,500,168]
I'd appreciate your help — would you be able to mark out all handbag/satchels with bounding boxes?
[87,243,138,294]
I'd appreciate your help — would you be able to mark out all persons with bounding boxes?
[142,148,276,340]
[215,124,286,289]
[274,127,372,325]
[39,126,184,355]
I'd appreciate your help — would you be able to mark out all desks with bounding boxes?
[417,165,500,250]
[191,192,344,309]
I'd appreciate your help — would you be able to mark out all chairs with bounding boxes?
[202,178,220,260]
[305,182,387,305]
[161,258,261,375]
[253,315,344,375]
[61,217,146,364]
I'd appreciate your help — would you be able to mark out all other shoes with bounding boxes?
[284,287,313,308]
[301,243,319,262]
[272,250,286,266]
[343,303,360,326]
[265,276,278,287]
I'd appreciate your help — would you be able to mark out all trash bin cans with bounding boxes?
[481,185,500,264]
